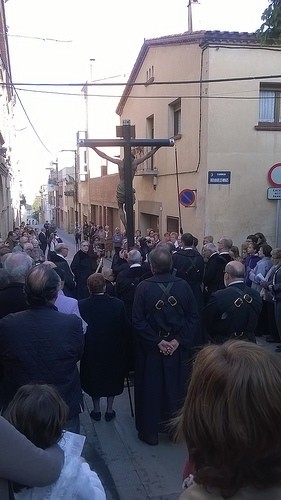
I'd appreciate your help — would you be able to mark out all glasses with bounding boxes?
[63,248,68,250]
[271,257,279,260]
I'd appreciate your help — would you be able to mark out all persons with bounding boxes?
[2,384,106,500]
[248,243,281,352]
[178,340,281,500]
[1,264,85,435]
[132,247,203,446]
[78,272,132,420]
[203,260,262,346]
[1,414,65,500]
[1,252,36,318]
[53,267,88,333]
[0,219,280,316]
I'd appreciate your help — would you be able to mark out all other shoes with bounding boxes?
[105,410,116,422]
[90,410,101,421]
[276,349,281,352]
[277,345,281,348]
[267,336,281,343]
[138,432,158,445]
